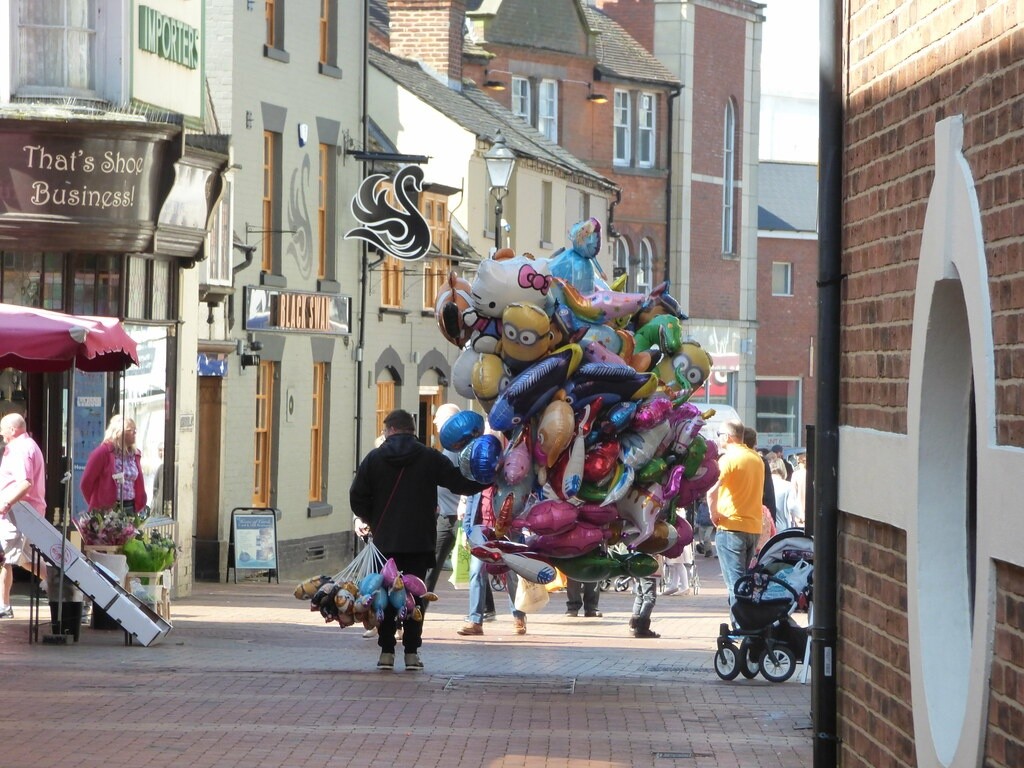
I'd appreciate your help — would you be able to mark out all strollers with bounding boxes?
[714,526,814,683]
[599,575,636,592]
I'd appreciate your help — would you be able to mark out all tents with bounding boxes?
[0,304,140,631]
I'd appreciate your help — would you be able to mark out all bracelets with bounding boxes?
[353,517,362,523]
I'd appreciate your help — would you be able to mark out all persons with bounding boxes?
[0,413,47,618]
[706,420,765,629]
[80,414,147,623]
[354,404,717,639]
[350,409,497,669]
[743,427,807,567]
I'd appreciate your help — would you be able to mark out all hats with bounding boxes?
[772,445,783,452]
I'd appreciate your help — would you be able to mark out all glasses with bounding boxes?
[125,427,137,434]
[717,431,727,437]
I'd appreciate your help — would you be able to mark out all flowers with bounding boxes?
[71,505,178,573]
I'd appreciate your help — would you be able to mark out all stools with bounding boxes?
[85,545,172,628]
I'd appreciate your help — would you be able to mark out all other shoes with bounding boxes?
[465,611,496,622]
[662,588,678,595]
[81,606,90,623]
[363,627,377,638]
[629,626,636,635]
[566,610,578,616]
[585,609,602,617]
[0,605,14,619]
[635,628,660,638]
[673,589,690,595]
[705,550,713,556]
[394,629,404,641]
[697,544,704,554]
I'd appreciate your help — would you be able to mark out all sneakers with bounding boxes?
[377,652,395,669]
[405,653,424,670]
[457,623,483,635]
[515,617,526,634]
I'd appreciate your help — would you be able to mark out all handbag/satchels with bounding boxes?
[761,559,812,599]
[448,526,471,589]
[514,573,549,613]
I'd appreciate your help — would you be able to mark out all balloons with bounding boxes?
[433,217,720,584]
[293,558,437,627]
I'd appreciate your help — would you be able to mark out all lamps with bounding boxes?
[562,79,608,104]
[483,68,509,90]
